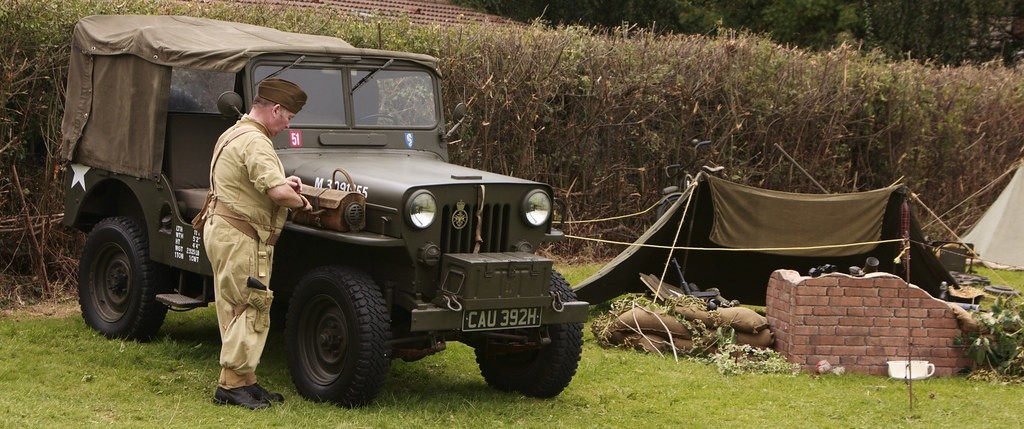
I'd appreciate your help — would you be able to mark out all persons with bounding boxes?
[203,79,313,411]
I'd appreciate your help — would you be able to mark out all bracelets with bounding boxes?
[296,194,307,211]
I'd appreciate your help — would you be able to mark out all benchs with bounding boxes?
[163,110,244,212]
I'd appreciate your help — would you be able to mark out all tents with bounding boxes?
[958,159,1024,271]
[572,171,980,308]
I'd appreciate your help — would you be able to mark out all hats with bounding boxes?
[258,78,307,114]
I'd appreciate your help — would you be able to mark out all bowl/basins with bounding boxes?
[886,360,935,380]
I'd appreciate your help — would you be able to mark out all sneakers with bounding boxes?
[213,386,271,410]
[242,383,284,406]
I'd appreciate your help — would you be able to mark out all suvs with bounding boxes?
[59,14,590,408]
